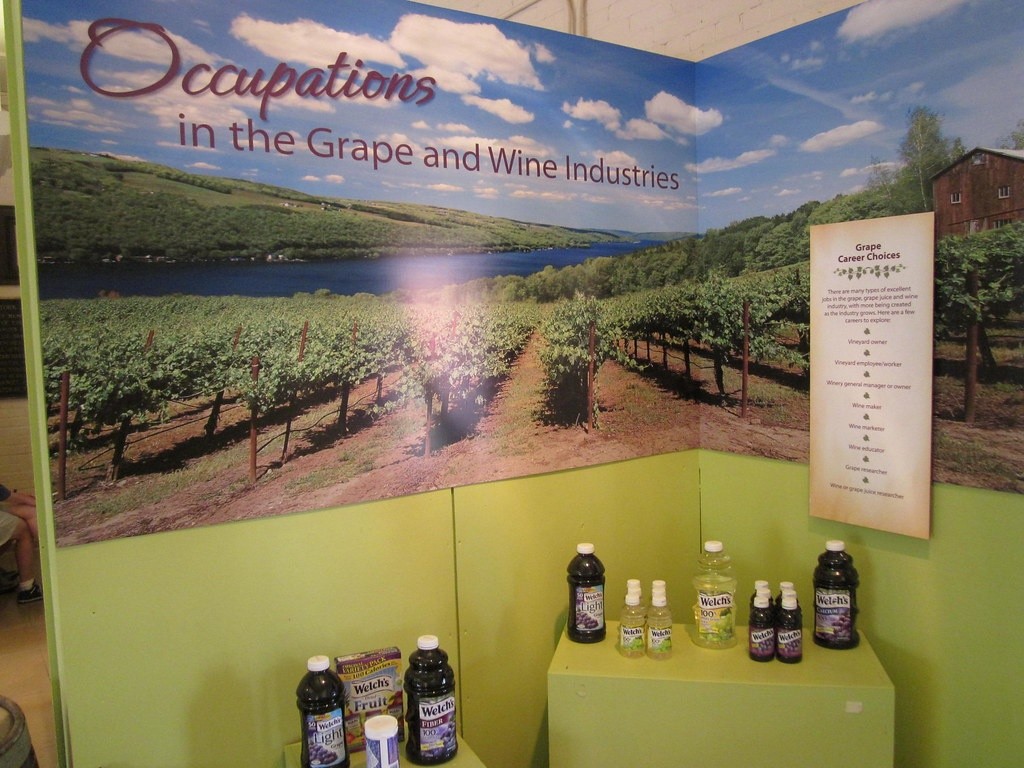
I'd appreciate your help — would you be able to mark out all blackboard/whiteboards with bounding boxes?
[0,298,27,398]
[0,204,20,285]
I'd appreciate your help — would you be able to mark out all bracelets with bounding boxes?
[14,489,17,493]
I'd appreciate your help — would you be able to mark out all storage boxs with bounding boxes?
[333,645,405,754]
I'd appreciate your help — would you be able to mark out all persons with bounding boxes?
[0,483,44,602]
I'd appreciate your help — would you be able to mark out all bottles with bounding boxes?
[403,635,458,765]
[691,541,737,650]
[749,580,803,663]
[566,542,606,643]
[812,539,860,650]
[620,579,672,659]
[296,655,350,768]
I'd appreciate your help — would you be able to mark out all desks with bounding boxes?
[548,620,896,768]
[283,720,485,768]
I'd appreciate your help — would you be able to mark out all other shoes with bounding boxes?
[0,568,18,579]
[0,577,19,594]
[17,582,43,603]
